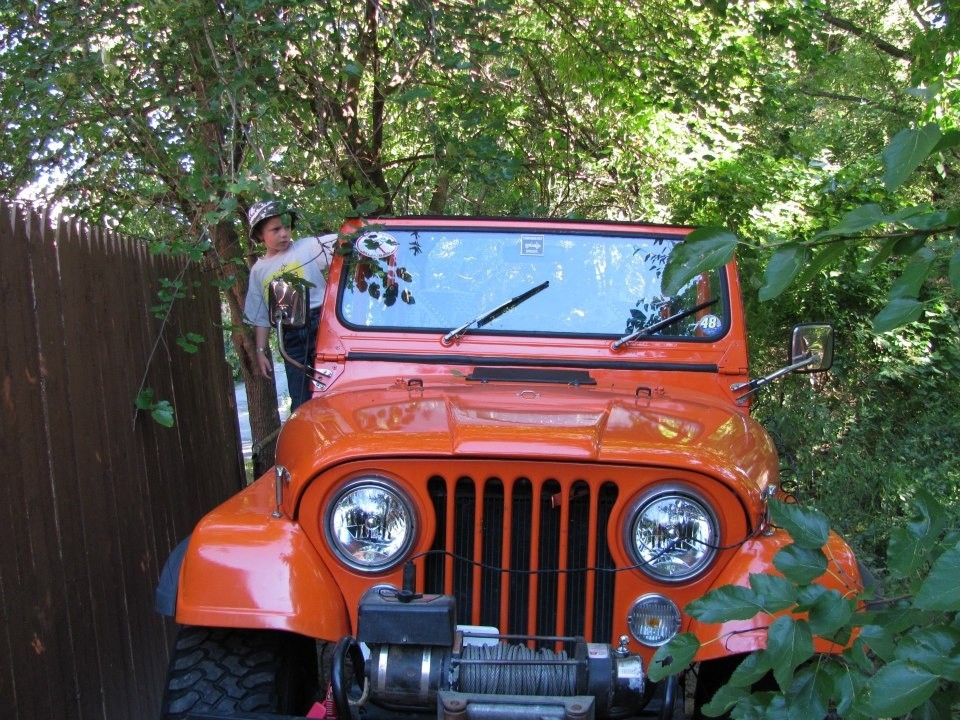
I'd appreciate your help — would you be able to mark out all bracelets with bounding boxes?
[256,347,265,354]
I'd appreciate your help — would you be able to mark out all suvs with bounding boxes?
[165,216,863,720]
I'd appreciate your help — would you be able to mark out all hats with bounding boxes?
[247,201,298,243]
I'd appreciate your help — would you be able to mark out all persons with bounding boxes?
[242,200,338,416]
